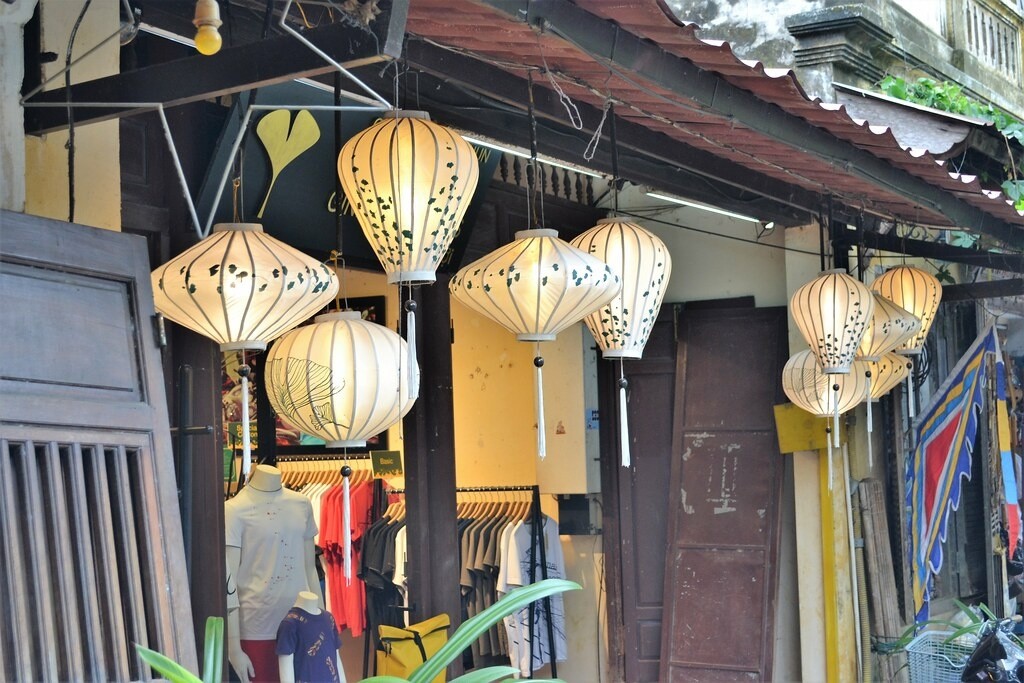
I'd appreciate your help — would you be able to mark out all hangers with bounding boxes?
[382,485,547,526]
[274,454,375,501]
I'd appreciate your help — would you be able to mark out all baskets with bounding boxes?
[905,631,979,683]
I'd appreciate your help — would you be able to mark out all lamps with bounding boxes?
[192,0,224,56]
